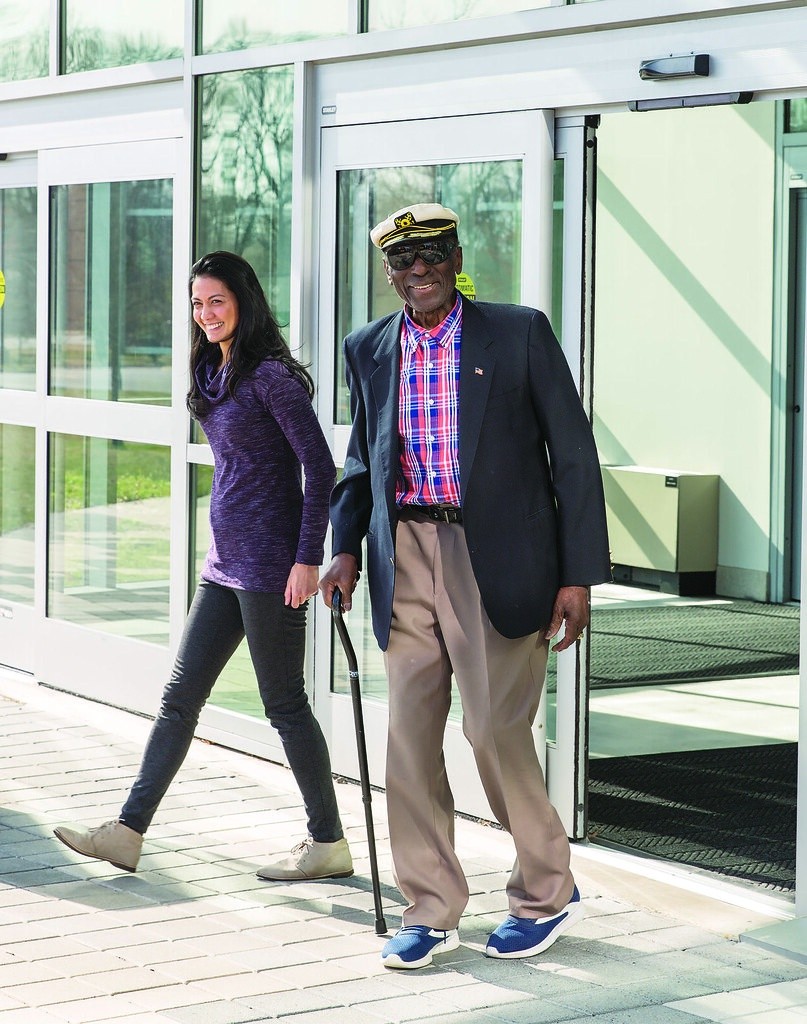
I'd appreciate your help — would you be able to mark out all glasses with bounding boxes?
[385,239,460,271]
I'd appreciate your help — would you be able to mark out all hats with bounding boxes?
[370,203,461,251]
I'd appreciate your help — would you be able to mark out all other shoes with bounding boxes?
[53,817,145,873]
[257,837,355,880]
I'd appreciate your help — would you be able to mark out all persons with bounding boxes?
[318,204,614,968]
[52,251,355,881]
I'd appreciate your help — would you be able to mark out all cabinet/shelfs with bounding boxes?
[602,465,720,597]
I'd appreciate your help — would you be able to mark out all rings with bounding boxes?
[577,633,584,640]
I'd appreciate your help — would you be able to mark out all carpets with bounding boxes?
[548,600,801,694]
[584,743,797,905]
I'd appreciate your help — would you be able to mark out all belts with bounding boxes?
[398,504,463,523]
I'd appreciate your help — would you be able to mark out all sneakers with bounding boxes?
[485,883,583,958]
[381,925,460,968]
